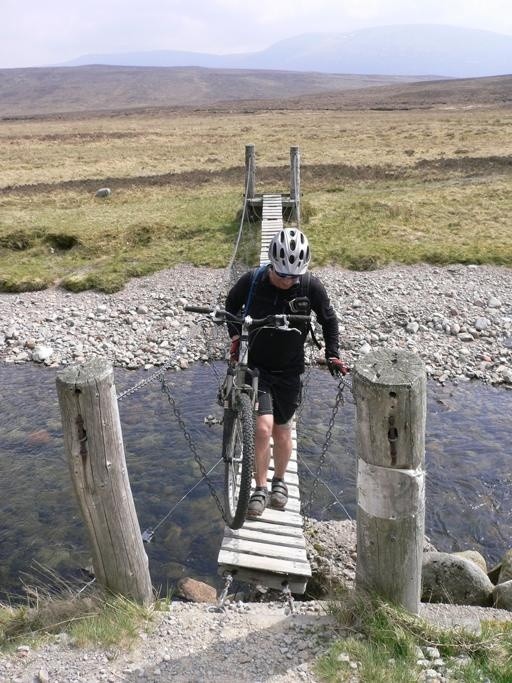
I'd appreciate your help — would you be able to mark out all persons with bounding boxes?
[225,228,346,515]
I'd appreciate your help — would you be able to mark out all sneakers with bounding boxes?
[246,490,269,520]
[270,478,288,508]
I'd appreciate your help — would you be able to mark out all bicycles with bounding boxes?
[183,293,311,529]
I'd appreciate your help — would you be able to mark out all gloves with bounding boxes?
[230,338,241,362]
[327,357,348,381]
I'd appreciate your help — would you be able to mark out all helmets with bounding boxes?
[267,227,312,275]
